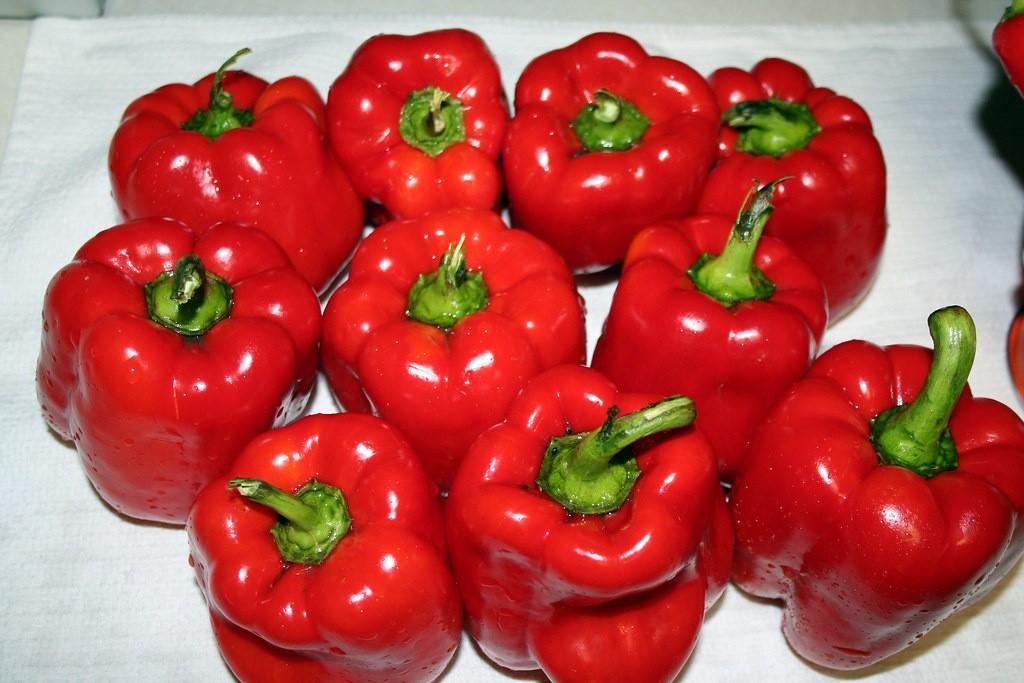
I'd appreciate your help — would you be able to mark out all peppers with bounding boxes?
[33,2,1024,683]
